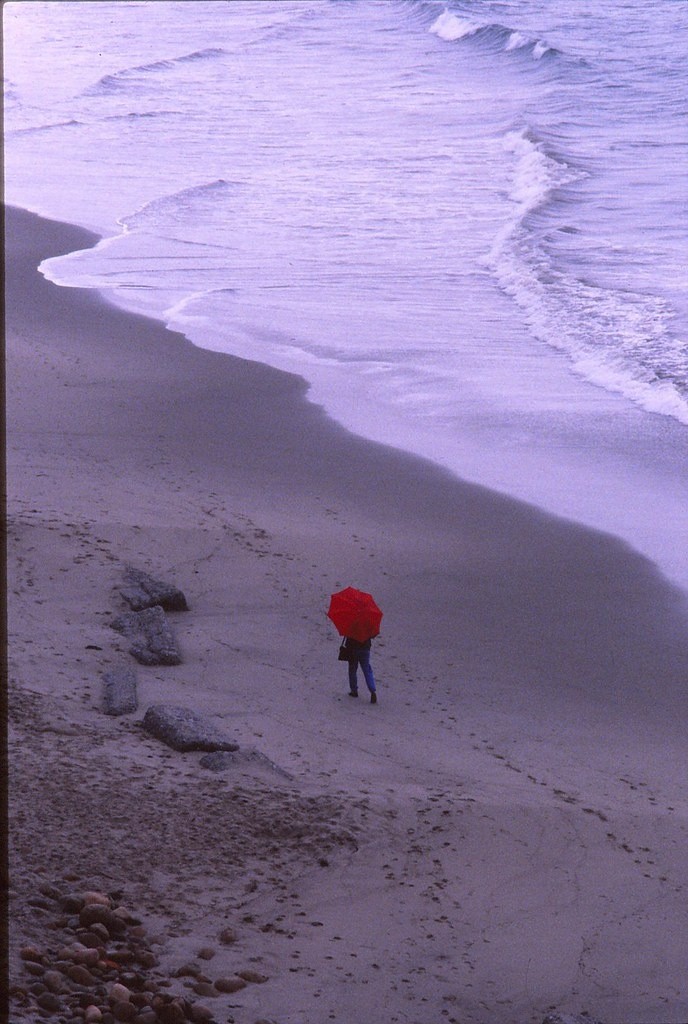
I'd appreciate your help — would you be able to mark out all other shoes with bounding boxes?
[370,692,377,703]
[348,691,358,697]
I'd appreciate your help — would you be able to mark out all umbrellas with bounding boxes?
[327,587,383,642]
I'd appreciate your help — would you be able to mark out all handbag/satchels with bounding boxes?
[338,646,349,661]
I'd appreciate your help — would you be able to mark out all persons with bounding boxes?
[345,637,376,703]
